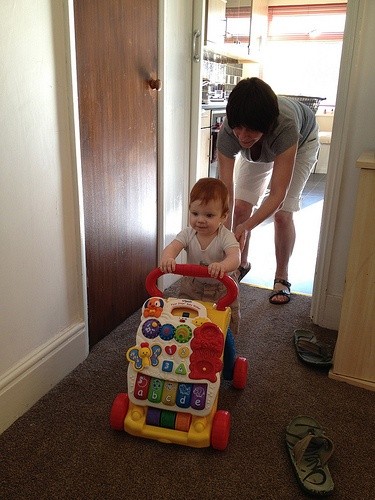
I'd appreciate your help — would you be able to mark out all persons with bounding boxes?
[217,77,321,305]
[159,178,242,340]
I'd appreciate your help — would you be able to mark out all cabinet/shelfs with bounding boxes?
[196,110,213,180]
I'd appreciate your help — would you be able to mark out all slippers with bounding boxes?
[234,262,251,284]
[294,328,333,365]
[285,415,335,493]
[269,277,293,304]
